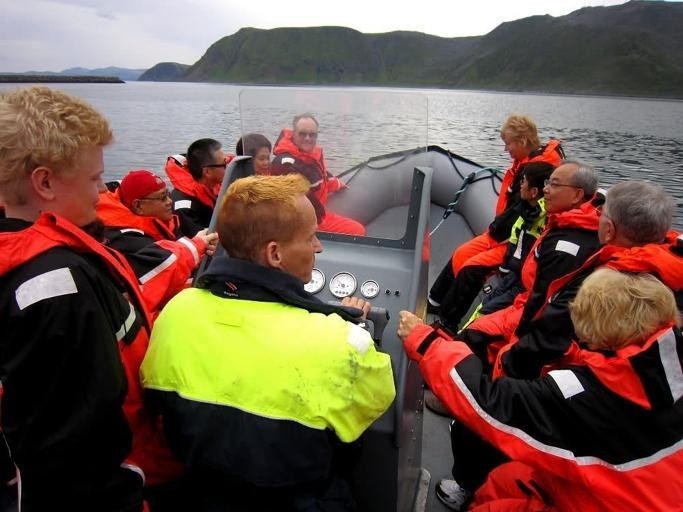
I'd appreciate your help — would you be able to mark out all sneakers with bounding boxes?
[423,393,455,421]
[433,476,470,511]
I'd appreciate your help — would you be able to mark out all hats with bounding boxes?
[118,170,166,207]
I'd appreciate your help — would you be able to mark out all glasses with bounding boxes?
[201,157,228,171]
[543,178,582,190]
[139,191,172,203]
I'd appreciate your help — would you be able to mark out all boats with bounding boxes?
[300,145,513,511]
